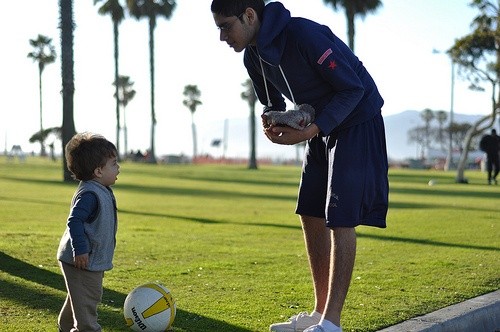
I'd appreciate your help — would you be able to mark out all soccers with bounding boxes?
[123,283,176,332]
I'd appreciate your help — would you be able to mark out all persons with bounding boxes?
[212,0,390,332]
[480,129,500,185]
[57,132,120,332]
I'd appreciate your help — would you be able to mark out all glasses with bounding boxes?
[217,12,244,33]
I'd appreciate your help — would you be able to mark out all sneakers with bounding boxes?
[269,310,318,332]
[300,324,343,331]
